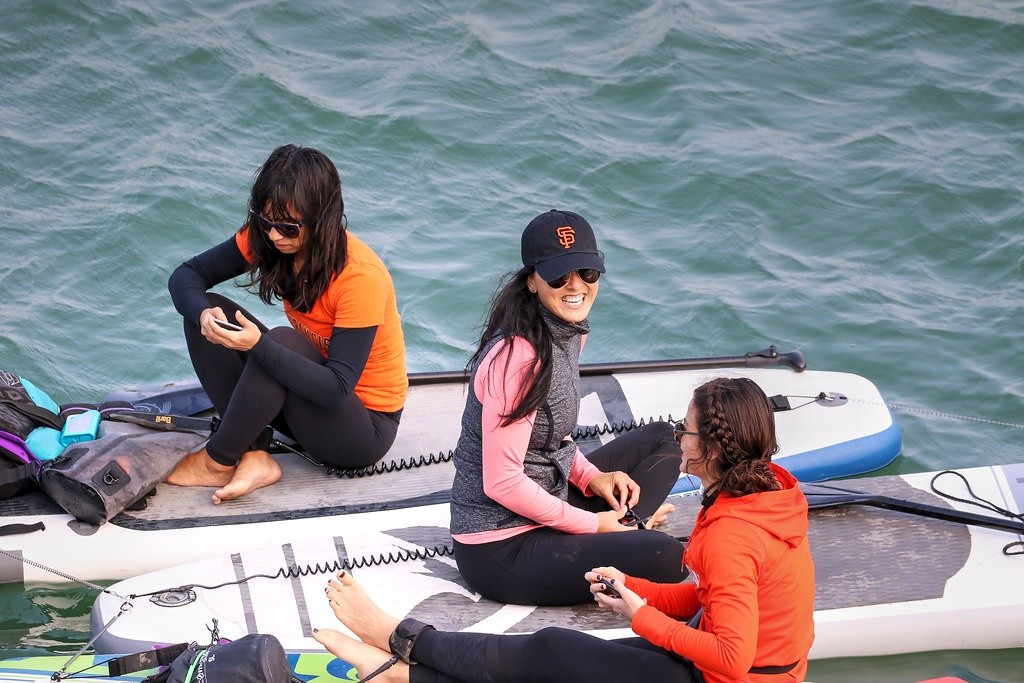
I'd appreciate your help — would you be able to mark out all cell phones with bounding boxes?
[624,516,652,526]
[600,576,621,598]
[214,318,243,331]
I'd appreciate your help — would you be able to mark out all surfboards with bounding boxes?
[0,363,899,586]
[87,457,1024,667]
[1,648,389,683]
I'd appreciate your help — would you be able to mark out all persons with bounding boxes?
[162,144,408,505]
[450,208,688,606]
[310,377,815,683]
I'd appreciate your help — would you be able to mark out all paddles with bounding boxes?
[798,478,1024,537]
[131,346,807,419]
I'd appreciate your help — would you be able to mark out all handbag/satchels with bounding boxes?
[141,634,293,683]
[0,368,210,526]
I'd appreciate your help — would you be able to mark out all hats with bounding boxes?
[521,209,606,281]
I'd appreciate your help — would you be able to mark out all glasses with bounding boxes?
[547,268,600,288]
[673,422,699,444]
[250,209,305,238]
